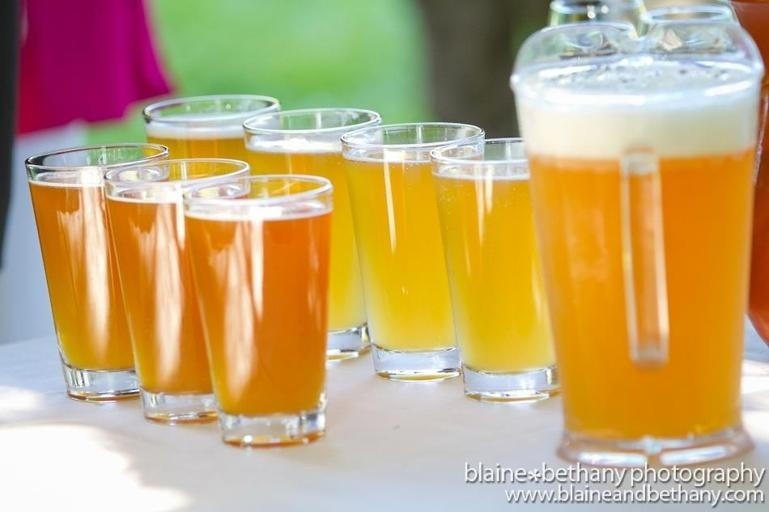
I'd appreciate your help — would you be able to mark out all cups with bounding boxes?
[25,3,763,466]
